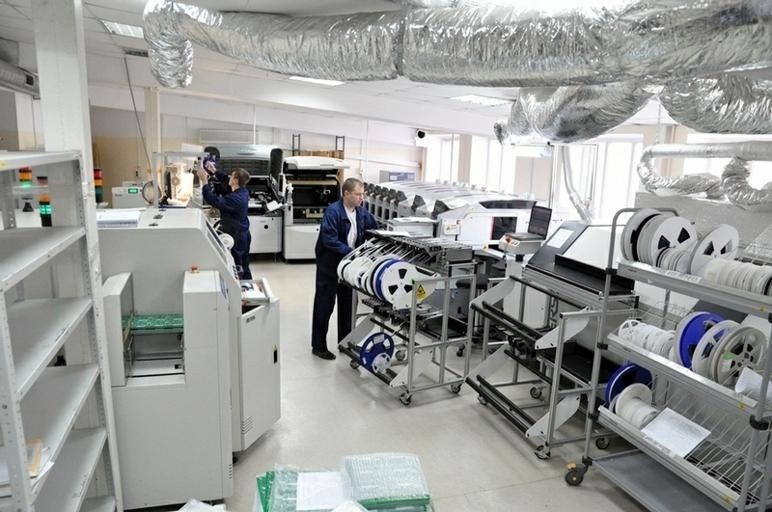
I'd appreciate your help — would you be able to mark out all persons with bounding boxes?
[311,178,378,360]
[197,162,252,280]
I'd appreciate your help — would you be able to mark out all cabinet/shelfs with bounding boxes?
[0,145,130,512]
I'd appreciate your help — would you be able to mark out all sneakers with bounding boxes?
[312,347,336,360]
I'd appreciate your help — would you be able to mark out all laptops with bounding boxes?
[505,205,552,240]
